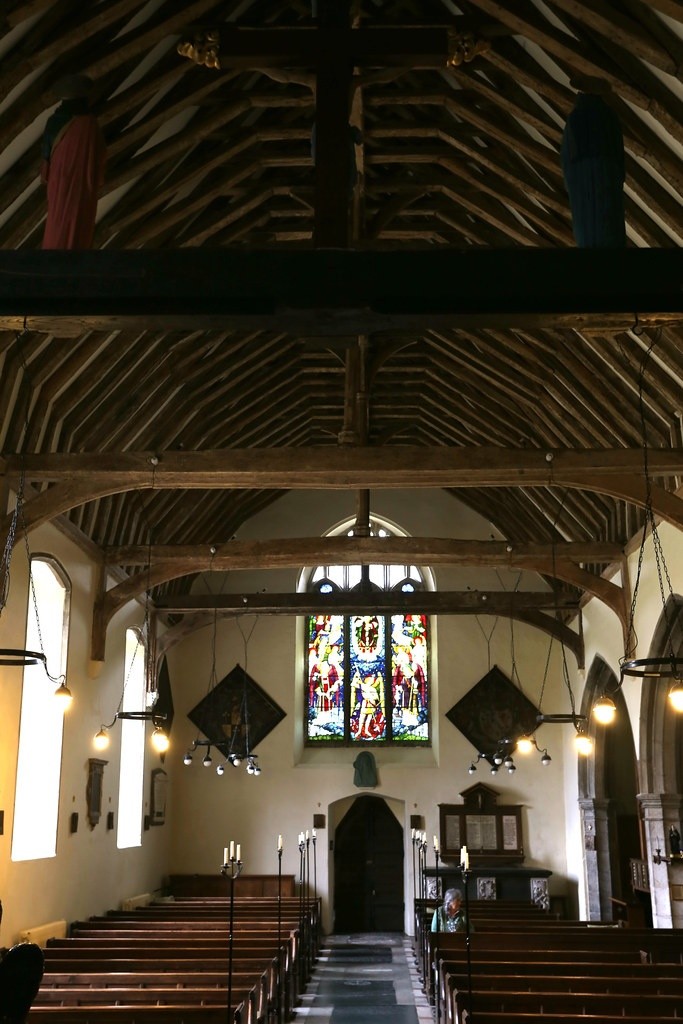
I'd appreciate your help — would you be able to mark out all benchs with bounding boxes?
[0,894,321,1024]
[414,897,683,1024]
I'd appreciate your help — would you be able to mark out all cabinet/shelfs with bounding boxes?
[170,874,297,898]
[423,867,553,900]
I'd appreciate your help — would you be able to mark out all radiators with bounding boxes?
[122,894,151,911]
[21,919,67,949]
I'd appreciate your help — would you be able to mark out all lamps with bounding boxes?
[467,331,683,776]
[0,314,74,710]
[184,570,261,777]
[94,490,170,755]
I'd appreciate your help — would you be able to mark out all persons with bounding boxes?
[432,889,476,933]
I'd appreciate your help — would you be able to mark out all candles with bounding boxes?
[278,835,282,850]
[410,829,428,846]
[460,846,469,870]
[433,835,439,850]
[223,840,241,863]
[298,828,317,843]
[656,836,659,849]
